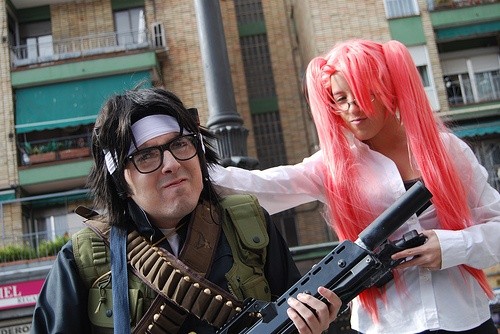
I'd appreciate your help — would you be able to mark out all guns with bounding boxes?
[212,180,434,334]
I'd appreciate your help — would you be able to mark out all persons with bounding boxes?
[30,80,343,334]
[207,40,500,334]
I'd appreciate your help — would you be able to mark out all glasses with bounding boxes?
[120,133,198,174]
[332,93,375,112]
